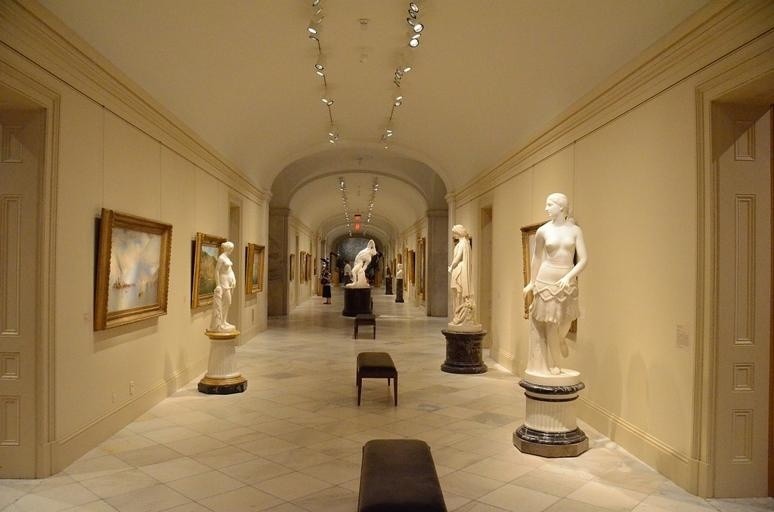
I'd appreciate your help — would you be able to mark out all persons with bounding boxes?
[446,224,479,325]
[521,192,588,376]
[210,241,236,333]
[343,258,353,286]
[320,267,331,305]
[395,263,403,277]
[345,239,376,287]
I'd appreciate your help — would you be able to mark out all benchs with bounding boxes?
[354,351,398,406]
[357,439,447,511]
[354,314,376,339]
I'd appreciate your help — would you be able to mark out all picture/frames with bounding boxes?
[94,207,266,330]
[402,238,426,301]
[520,217,577,333]
[290,251,317,285]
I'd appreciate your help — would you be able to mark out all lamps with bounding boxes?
[405,1,425,49]
[308,21,334,108]
[392,62,412,109]
